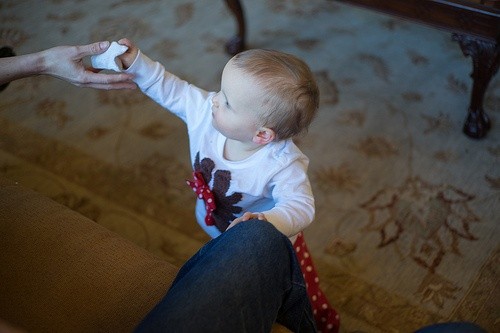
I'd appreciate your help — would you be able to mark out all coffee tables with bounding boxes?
[223,0,499,140]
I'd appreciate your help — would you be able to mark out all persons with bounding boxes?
[126,219,488,333]
[0,39,137,90]
[115,37,344,332]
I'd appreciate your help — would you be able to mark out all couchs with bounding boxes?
[0,174,293,333]
